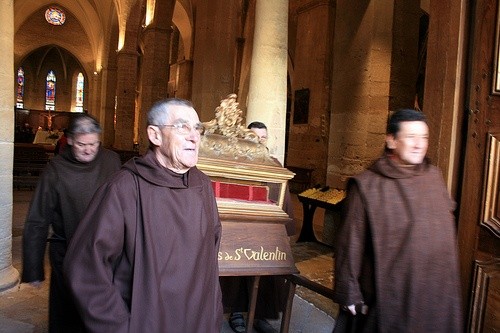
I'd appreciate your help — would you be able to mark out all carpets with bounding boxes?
[219,293,336,333]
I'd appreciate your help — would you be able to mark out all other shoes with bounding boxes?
[229,312,246,332]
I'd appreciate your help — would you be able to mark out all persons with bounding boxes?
[62,98,223,333]
[19,115,122,333]
[229,122,295,333]
[333,110,463,333]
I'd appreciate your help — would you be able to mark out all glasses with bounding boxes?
[153,122,207,135]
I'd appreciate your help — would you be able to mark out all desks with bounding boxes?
[33,131,63,145]
